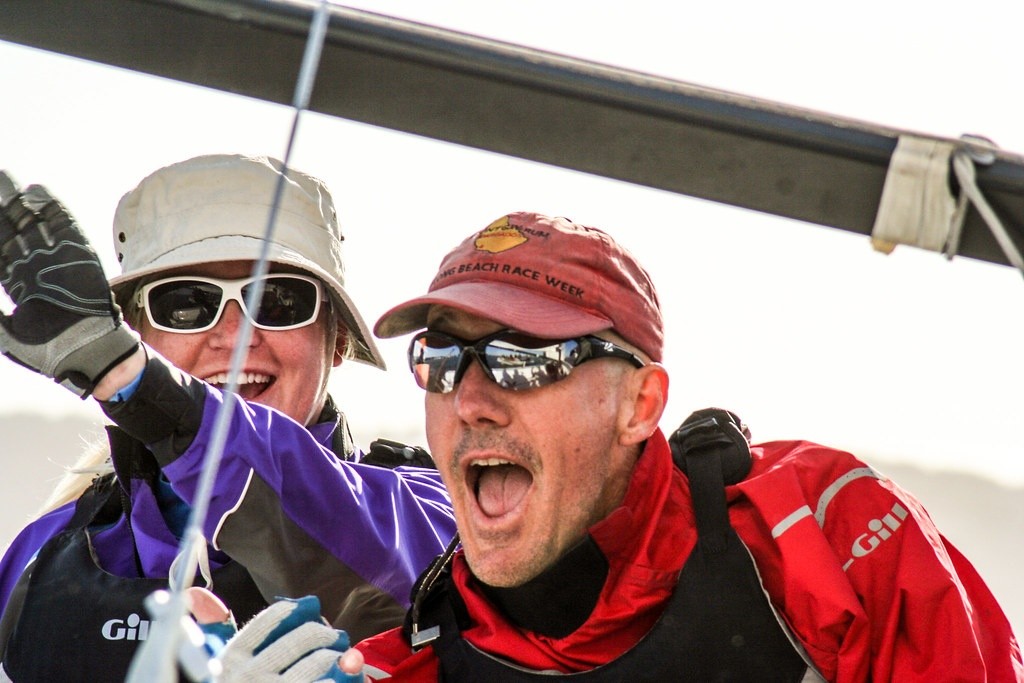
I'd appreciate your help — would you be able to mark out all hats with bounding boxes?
[105,153,389,371]
[373,210,664,363]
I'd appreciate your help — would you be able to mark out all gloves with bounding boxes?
[54,197,141,402]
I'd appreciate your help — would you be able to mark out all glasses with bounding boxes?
[406,325,647,395]
[131,272,330,333]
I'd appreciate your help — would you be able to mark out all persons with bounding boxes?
[0,153,462,683]
[185,211,1024,683]
